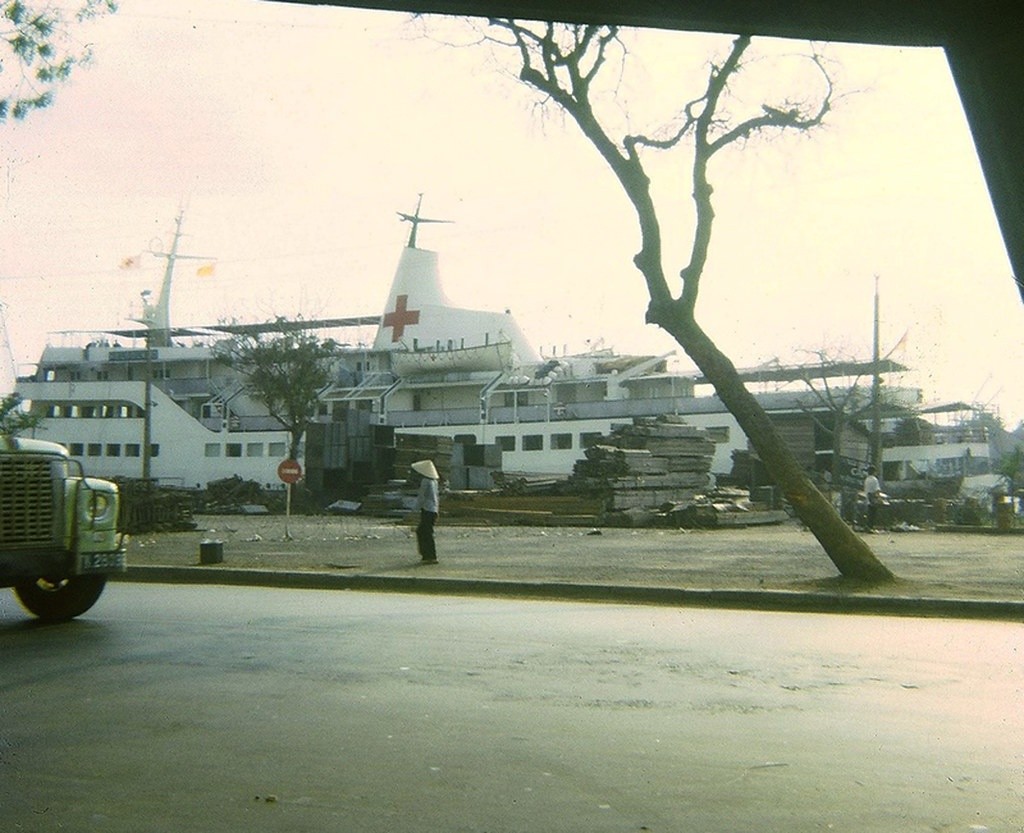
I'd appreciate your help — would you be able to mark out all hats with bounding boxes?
[411,460,440,480]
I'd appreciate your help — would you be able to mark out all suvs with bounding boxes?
[1,435,129,622]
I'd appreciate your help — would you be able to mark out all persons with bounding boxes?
[863,467,880,532]
[412,461,440,563]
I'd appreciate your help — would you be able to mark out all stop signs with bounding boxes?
[277,460,302,484]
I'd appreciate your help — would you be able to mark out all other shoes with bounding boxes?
[868,529,879,534]
[419,559,438,564]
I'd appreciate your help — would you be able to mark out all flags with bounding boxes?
[197,259,216,275]
[117,253,141,284]
